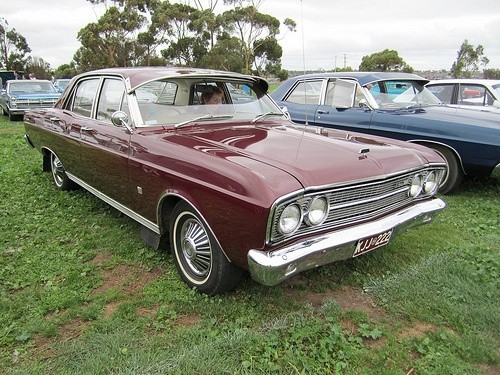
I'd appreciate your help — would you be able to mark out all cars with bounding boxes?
[21,67,447,300]
[268,70,500,194]
[0,78,255,123]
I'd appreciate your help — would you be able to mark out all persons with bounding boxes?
[192,84,225,115]
[13,69,22,80]
[30,73,36,80]
